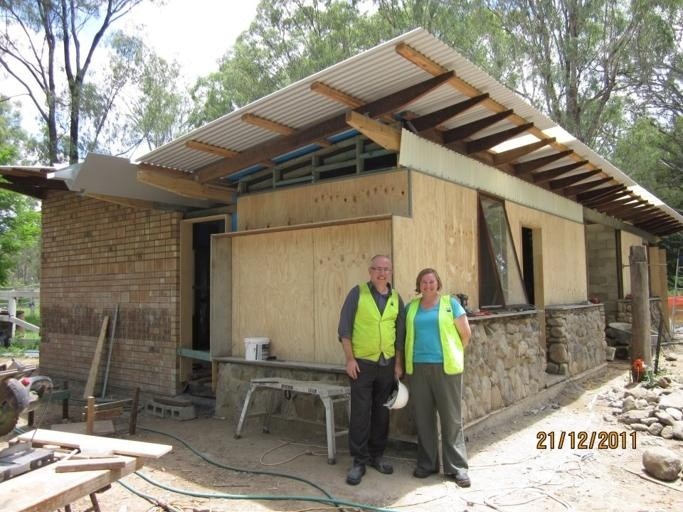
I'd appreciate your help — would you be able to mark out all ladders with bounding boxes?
[671,246,683,340]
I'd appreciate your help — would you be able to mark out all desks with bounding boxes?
[0,424,172,510]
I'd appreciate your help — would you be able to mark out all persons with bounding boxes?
[337,254,406,487]
[405,267,470,486]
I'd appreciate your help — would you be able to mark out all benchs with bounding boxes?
[231,377,352,464]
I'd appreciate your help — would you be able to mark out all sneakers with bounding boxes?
[413,468,431,478]
[347,457,394,485]
[453,474,471,487]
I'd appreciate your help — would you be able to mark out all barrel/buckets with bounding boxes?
[242,335,272,362]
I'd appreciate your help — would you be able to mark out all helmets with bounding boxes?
[387,380,409,410]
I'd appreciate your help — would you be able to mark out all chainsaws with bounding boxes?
[1,374,57,482]
